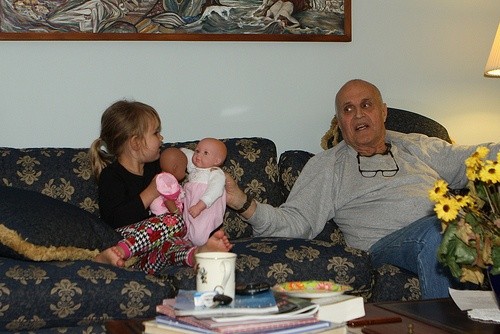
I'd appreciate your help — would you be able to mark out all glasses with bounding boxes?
[357,152,399,179]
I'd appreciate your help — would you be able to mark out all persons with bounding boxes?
[180,138,227,246]
[149,148,187,216]
[226,80,500,300]
[90,101,234,274]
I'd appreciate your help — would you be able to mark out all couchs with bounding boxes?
[0,107,420,334]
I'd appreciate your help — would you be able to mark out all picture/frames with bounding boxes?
[0,0,353,43]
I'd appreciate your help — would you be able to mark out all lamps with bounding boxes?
[484,24,500,79]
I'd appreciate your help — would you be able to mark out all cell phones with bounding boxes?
[236,282,270,295]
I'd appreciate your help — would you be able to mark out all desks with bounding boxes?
[106,294,500,334]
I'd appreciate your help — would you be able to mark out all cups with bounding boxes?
[195,252,237,306]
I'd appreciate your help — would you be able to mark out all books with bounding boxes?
[155,288,331,334]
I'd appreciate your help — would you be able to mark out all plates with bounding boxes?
[272,283,354,298]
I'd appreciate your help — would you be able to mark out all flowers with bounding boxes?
[428,145,500,289]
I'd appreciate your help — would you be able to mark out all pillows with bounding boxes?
[0,185,120,262]
[320,108,453,150]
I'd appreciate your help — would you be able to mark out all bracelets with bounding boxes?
[236,193,251,213]
